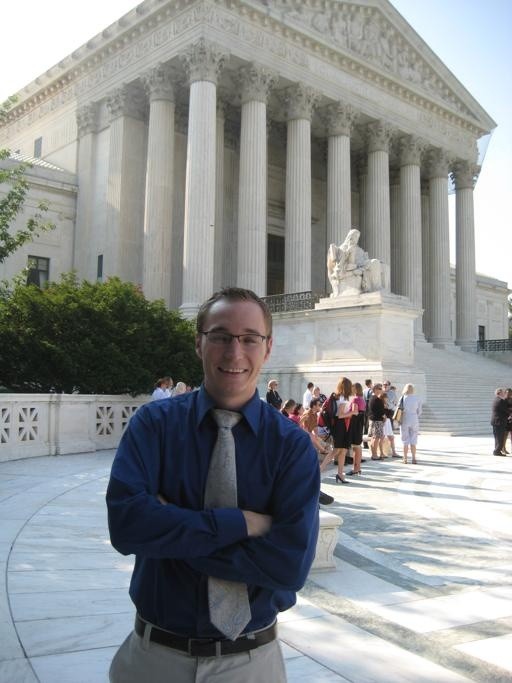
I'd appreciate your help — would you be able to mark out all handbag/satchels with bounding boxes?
[391,397,404,422]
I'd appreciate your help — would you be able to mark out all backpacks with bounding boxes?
[318,392,338,433]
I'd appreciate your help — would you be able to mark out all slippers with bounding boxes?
[346,470,361,475]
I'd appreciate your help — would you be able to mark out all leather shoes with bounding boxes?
[371,454,417,464]
[502,448,509,454]
[494,452,506,456]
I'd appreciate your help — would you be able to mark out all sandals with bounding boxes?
[320,449,329,454]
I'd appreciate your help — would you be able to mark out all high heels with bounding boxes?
[335,474,350,483]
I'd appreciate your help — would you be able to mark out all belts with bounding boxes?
[136,611,277,657]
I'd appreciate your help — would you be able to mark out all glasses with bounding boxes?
[202,330,267,350]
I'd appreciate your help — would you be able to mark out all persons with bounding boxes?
[490,388,512,456]
[266,377,422,483]
[151,376,206,400]
[107,288,320,683]
[330,229,384,293]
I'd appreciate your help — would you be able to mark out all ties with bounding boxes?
[202,408,251,641]
